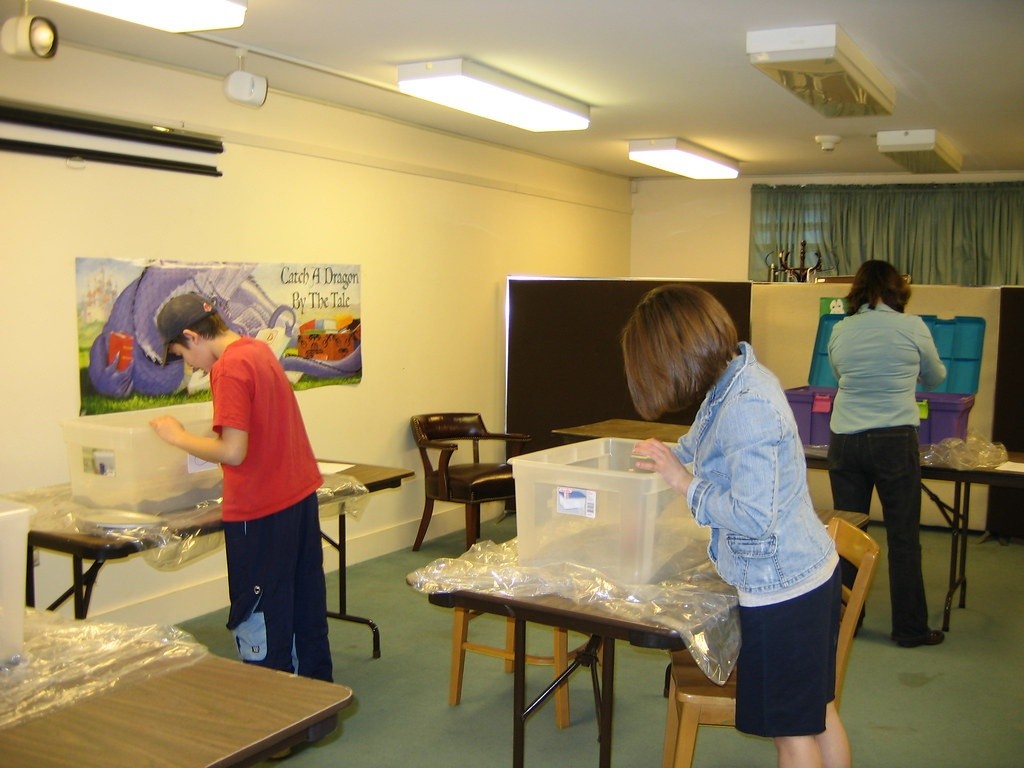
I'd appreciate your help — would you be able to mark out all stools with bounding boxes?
[447,603,604,730]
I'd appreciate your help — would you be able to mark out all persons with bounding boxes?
[148,294,335,759]
[827,259,946,648]
[619,280,853,768]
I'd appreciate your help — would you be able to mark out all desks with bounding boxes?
[25,459,416,660]
[0,612,356,768]
[404,509,870,768]
[552,417,692,443]
[806,453,1024,634]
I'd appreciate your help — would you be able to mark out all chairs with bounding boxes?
[662,516,881,768]
[410,411,535,552]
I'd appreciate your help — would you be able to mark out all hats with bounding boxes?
[158,293,217,366]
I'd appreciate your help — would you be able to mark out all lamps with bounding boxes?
[222,48,269,109]
[627,137,740,180]
[747,23,897,120]
[0,0,59,62]
[396,57,592,133]
[54,0,249,35]
[876,129,964,175]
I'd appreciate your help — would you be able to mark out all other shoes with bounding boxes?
[899,627,944,647]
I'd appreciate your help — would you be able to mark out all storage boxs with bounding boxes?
[58,403,223,515]
[507,436,712,587]
[0,495,38,659]
[783,385,976,451]
